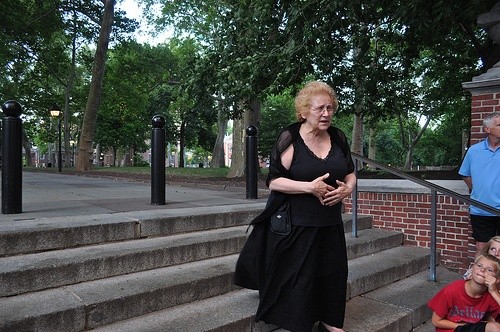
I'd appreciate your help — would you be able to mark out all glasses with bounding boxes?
[310,104,336,114]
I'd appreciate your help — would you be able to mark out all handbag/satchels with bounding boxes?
[270,201,292,236]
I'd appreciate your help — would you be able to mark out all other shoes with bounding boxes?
[317,322,331,332]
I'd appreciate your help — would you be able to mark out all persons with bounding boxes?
[427,252,500,332]
[232,80,358,332]
[458,111,500,258]
[480,235,500,262]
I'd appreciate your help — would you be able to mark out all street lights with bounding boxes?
[50,104,62,171]
